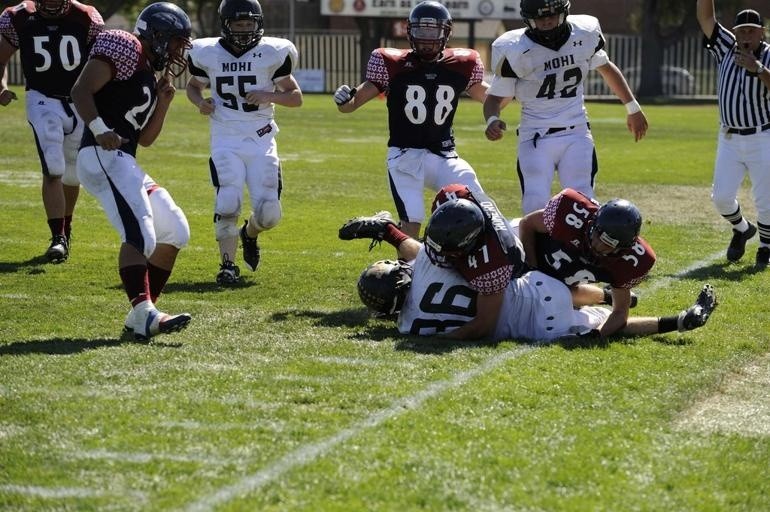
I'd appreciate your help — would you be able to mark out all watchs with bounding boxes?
[752,64,764,77]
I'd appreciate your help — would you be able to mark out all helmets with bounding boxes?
[586,199,642,258]
[357,258,414,314]
[217,0,264,48]
[423,198,485,269]
[133,1,193,76]
[520,0,571,39]
[407,1,453,59]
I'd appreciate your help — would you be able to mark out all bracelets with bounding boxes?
[87,117,115,139]
[484,115,500,132]
[625,100,642,115]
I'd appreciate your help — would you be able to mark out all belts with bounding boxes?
[728,123,770,135]
[534,126,574,147]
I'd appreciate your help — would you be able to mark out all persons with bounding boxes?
[483,1,650,219]
[0,0,107,264]
[358,241,718,342]
[697,0,769,268]
[335,1,526,261]
[186,0,304,286]
[339,184,527,345]
[341,187,656,346]
[70,2,193,348]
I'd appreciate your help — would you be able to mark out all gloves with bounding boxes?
[334,84,356,105]
[572,329,605,349]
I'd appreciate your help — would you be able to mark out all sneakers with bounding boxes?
[338,210,397,241]
[239,224,261,272]
[678,283,716,332]
[47,235,70,258]
[756,247,770,264]
[124,308,191,341]
[217,260,241,284]
[727,221,757,261]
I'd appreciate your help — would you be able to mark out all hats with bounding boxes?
[732,9,766,29]
